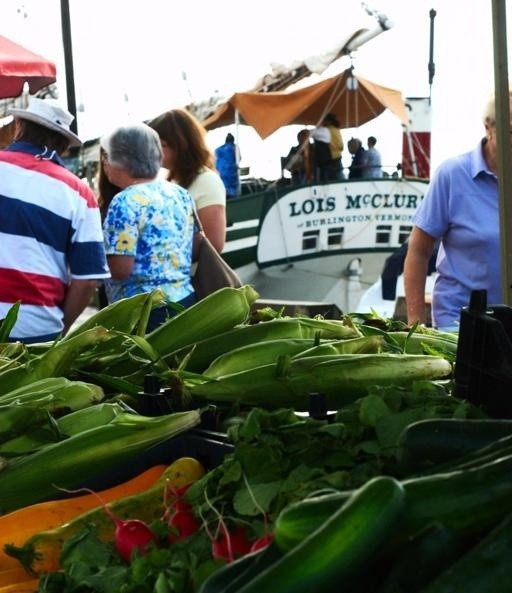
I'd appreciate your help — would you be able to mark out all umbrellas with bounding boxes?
[0,33,57,99]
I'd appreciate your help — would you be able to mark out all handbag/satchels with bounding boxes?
[191,231,243,300]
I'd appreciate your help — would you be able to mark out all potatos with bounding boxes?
[47,479,274,565]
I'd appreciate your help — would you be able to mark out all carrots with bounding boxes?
[0,455,204,592]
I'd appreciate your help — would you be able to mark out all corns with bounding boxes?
[0,283,460,510]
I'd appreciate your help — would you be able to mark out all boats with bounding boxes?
[89,6,440,318]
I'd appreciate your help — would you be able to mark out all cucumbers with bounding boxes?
[197,419,510,592]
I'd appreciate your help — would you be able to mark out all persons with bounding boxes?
[98,145,124,309]
[347,137,364,179]
[214,133,241,197]
[312,113,345,181]
[0,96,112,354]
[402,87,512,332]
[286,128,314,184]
[98,120,196,332]
[360,136,384,180]
[150,108,227,280]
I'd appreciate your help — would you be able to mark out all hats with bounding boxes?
[8,96,83,147]
